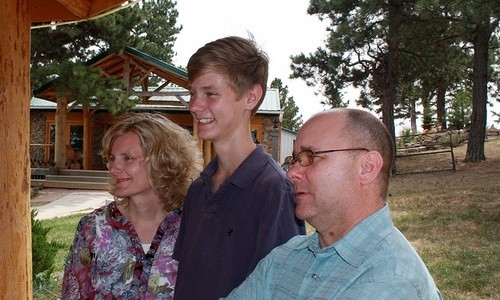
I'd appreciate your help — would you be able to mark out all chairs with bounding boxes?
[65,144,82,169]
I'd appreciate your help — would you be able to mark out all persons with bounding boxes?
[60,110,206,300]
[434,121,447,150]
[172,34,308,300]
[421,125,439,150]
[224,107,443,300]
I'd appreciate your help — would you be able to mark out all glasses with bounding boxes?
[283,146,370,169]
